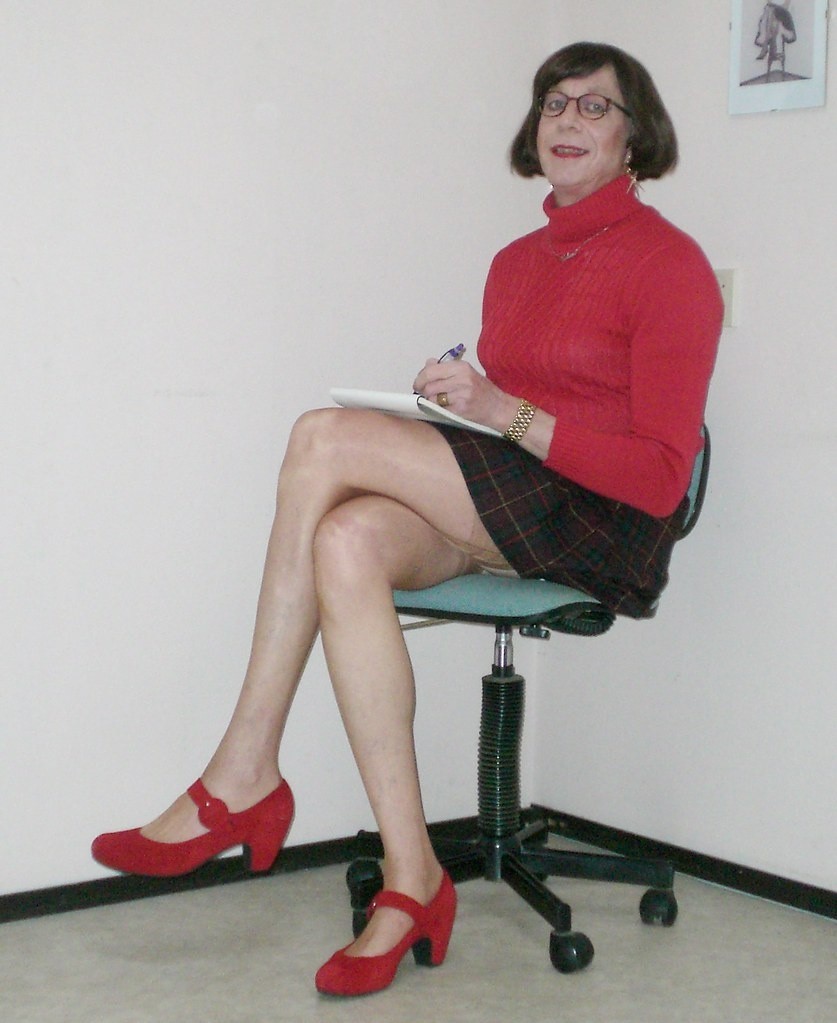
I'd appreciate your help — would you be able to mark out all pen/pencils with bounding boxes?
[413,343,464,394]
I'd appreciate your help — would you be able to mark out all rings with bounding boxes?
[437,392,449,406]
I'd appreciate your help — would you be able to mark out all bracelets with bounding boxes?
[502,396,537,443]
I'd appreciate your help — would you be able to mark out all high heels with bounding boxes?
[91,774,294,876]
[314,868,457,994]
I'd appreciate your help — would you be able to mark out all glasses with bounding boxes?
[538,91,633,121]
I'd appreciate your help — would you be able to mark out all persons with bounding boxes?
[92,43,725,996]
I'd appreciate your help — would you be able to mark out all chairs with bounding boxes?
[348,409,712,973]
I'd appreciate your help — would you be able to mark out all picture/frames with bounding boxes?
[728,0,829,117]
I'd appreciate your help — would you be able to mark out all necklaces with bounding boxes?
[553,225,611,261]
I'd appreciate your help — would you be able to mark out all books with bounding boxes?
[330,388,503,439]
